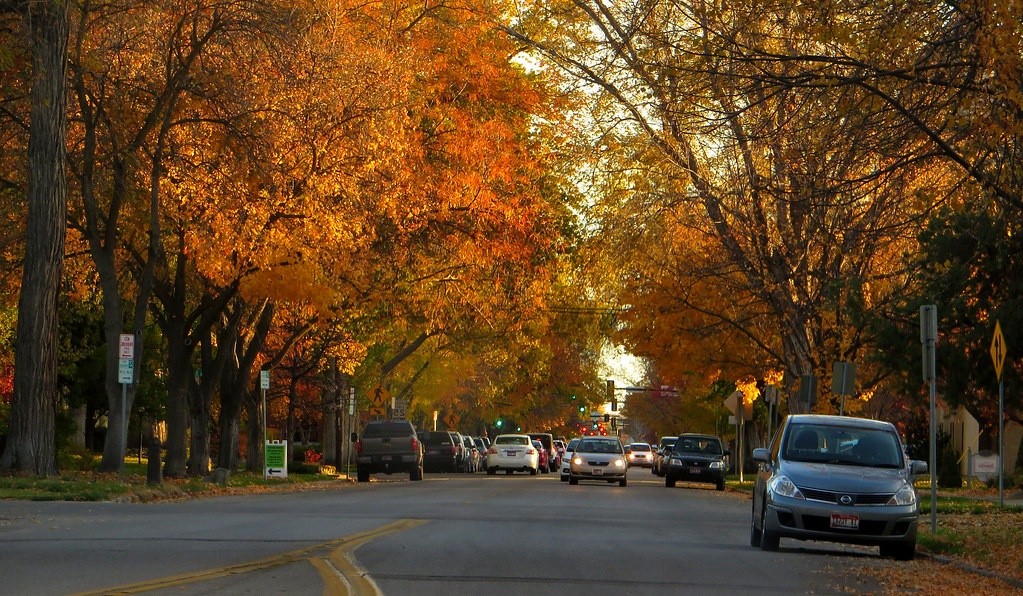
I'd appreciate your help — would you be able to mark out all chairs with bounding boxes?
[585,444,594,450]
[852,443,875,460]
[794,430,819,454]
[702,443,717,453]
[682,442,692,451]
[608,444,616,451]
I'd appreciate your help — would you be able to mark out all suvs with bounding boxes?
[352,419,426,482]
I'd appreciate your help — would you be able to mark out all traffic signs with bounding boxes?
[119,359,134,384]
[516,426,521,432]
[496,418,504,428]
[118,333,135,359]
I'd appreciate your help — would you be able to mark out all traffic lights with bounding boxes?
[579,404,586,414]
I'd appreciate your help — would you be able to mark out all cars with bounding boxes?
[530,440,550,474]
[665,433,730,491]
[751,415,931,560]
[462,435,491,472]
[628,443,654,468]
[449,431,467,473]
[651,437,692,476]
[487,434,538,474]
[527,433,569,472]
[571,436,631,487]
[416,431,459,472]
[558,439,581,481]
